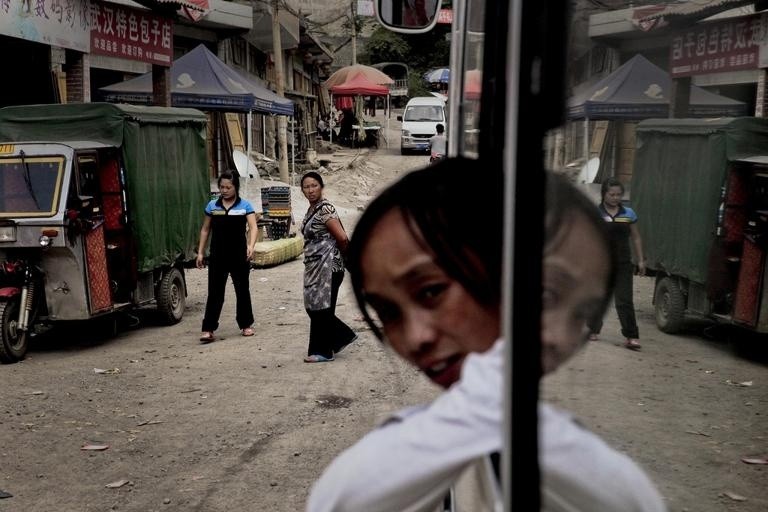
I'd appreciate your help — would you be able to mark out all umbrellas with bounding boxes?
[321,65,396,117]
[422,66,450,90]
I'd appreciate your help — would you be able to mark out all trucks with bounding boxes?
[366,62,411,108]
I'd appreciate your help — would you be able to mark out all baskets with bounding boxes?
[261,186,291,240]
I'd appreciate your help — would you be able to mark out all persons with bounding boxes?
[196,171,258,341]
[303,156,503,511]
[301,171,358,361]
[318,115,328,137]
[540,172,668,512]
[427,124,447,160]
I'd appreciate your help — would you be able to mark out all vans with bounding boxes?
[397,97,446,156]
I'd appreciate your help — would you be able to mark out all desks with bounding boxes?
[351,125,381,148]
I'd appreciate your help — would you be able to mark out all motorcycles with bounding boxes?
[626,114,768,339]
[1,102,213,362]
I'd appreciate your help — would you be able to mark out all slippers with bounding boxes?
[304,336,358,362]
[625,339,642,350]
[243,327,254,336]
[200,331,216,341]
[589,334,599,342]
